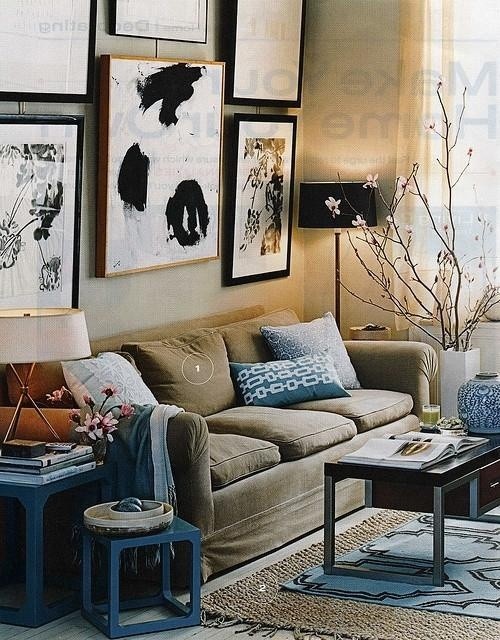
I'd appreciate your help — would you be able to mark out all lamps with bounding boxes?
[0,305,100,447]
[295,178,380,331]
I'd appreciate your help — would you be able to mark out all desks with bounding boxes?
[0,461,111,632]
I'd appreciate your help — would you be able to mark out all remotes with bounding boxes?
[45,441,77,451]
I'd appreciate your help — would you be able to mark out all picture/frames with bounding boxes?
[106,0,210,45]
[224,0,311,111]
[217,112,299,289]
[0,113,88,322]
[89,53,227,278]
[0,0,98,104]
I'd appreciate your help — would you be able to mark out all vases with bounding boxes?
[75,436,110,467]
[454,371,500,440]
[434,344,479,428]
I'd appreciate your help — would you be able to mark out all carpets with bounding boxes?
[275,510,500,624]
[190,504,500,640]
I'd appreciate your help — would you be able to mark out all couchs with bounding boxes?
[0,302,440,590]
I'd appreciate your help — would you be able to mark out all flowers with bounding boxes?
[44,381,141,445]
[320,75,499,352]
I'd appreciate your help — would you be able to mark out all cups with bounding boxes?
[423,405,440,424]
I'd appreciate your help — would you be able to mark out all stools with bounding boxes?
[77,512,207,640]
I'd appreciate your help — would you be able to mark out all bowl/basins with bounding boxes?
[109,501,165,521]
[84,500,174,537]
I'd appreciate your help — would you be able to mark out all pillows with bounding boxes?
[227,343,353,409]
[257,310,365,391]
[56,349,161,412]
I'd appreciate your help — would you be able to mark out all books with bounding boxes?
[336,430,493,471]
[0,444,97,486]
